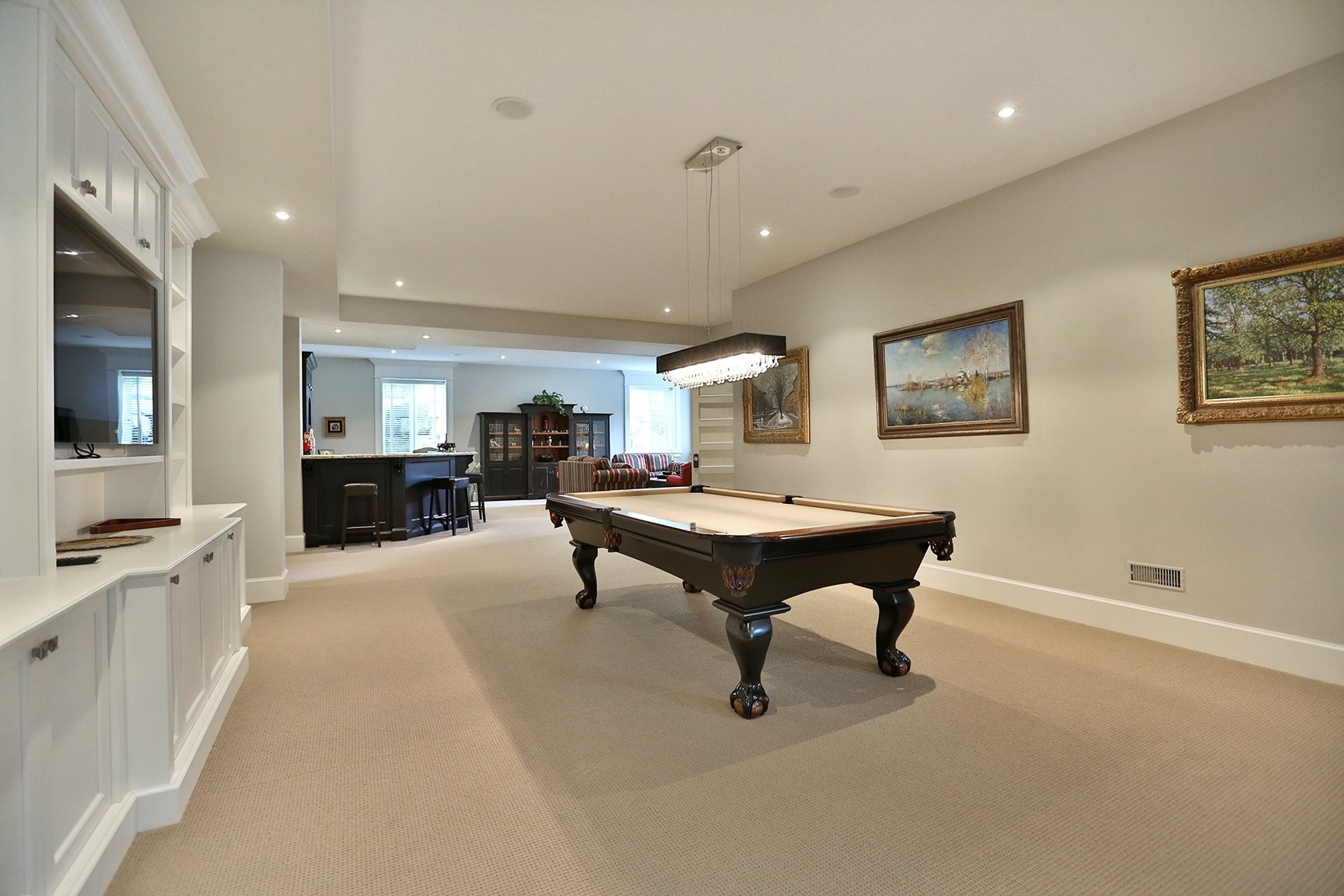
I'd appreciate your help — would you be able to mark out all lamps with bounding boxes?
[657,137,789,390]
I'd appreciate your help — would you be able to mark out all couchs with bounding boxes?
[611,453,686,477]
[666,462,692,487]
[557,460,650,489]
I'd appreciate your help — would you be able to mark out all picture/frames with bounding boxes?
[741,345,810,445]
[1170,236,1344,425]
[323,416,347,437]
[871,299,1029,440]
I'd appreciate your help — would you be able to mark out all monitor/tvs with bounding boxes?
[53,202,157,447]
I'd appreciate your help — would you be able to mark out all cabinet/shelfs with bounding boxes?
[170,225,187,462]
[168,527,236,760]
[49,37,164,268]
[475,403,614,500]
[0,576,112,896]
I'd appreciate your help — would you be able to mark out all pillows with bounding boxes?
[611,462,633,468]
[581,456,613,470]
[566,455,586,461]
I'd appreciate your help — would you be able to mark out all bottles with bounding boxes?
[555,422,558,431]
[303,432,311,455]
[309,428,315,455]
[543,416,548,431]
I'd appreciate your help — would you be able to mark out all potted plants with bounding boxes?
[533,389,564,404]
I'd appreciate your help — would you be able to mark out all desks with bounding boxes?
[546,484,958,720]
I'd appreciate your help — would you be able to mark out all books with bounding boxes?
[489,423,522,461]
[576,424,600,458]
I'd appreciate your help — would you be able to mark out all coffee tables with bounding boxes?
[650,477,667,487]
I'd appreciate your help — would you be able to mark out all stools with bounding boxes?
[340,483,381,550]
[428,476,474,536]
[457,472,486,524]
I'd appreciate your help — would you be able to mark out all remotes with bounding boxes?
[57,554,102,567]
